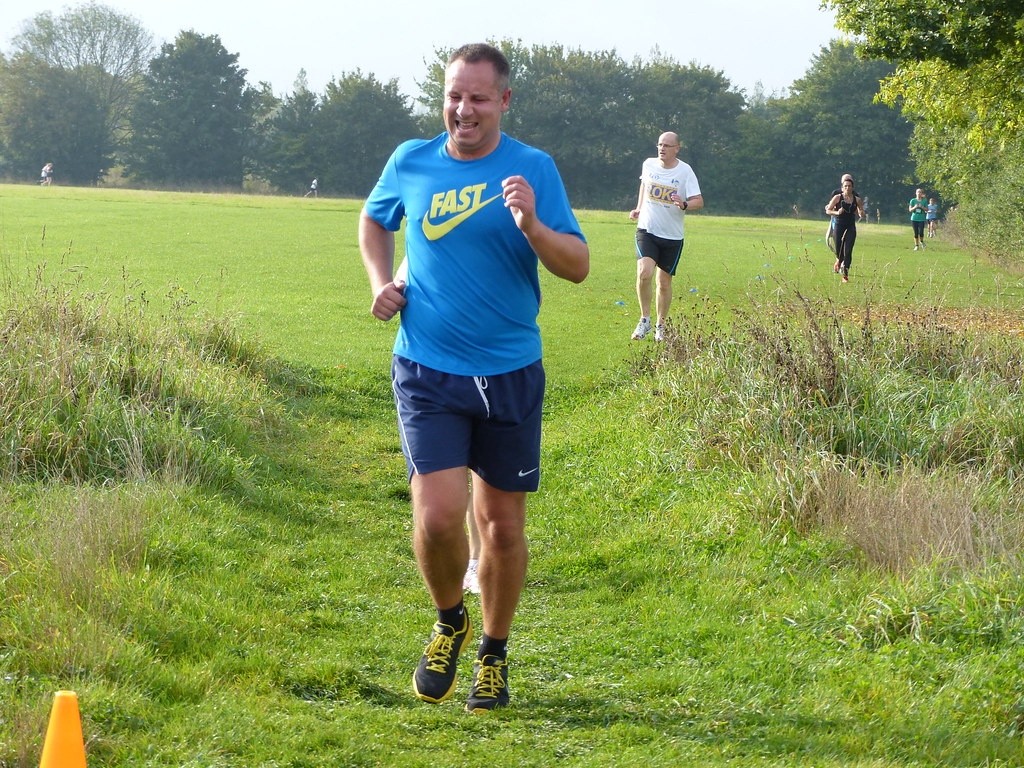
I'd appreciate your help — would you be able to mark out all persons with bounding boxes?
[925,197,938,238]
[303,176,319,198]
[825,173,864,285]
[40,161,54,186]
[356,42,591,720]
[907,187,929,252]
[857,195,869,223]
[629,129,706,342]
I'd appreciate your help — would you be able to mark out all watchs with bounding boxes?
[680,201,689,210]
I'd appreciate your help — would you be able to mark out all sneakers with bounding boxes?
[411,605,474,704]
[654,323,668,341]
[842,273,849,283]
[464,653,512,716]
[913,245,919,251]
[630,321,652,340]
[927,233,936,238]
[920,241,926,247]
[834,261,844,275]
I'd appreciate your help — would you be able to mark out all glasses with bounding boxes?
[655,142,677,149]
